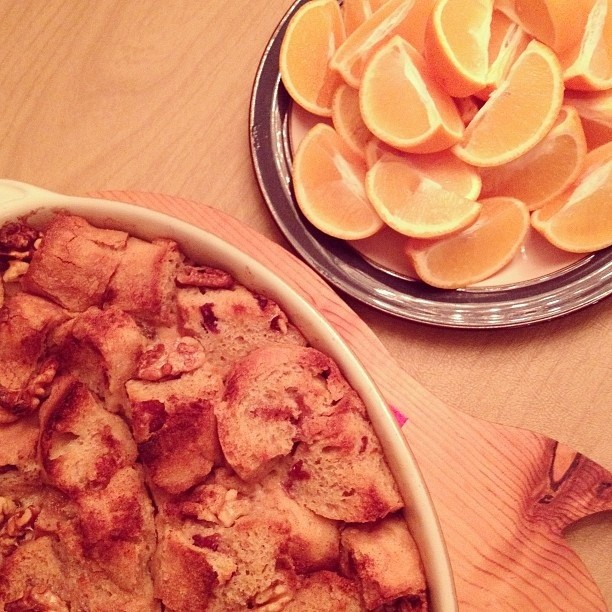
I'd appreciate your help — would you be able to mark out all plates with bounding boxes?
[1,179,459,612]
[251,1,612,330]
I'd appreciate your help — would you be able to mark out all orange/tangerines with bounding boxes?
[279,0,612,292]
